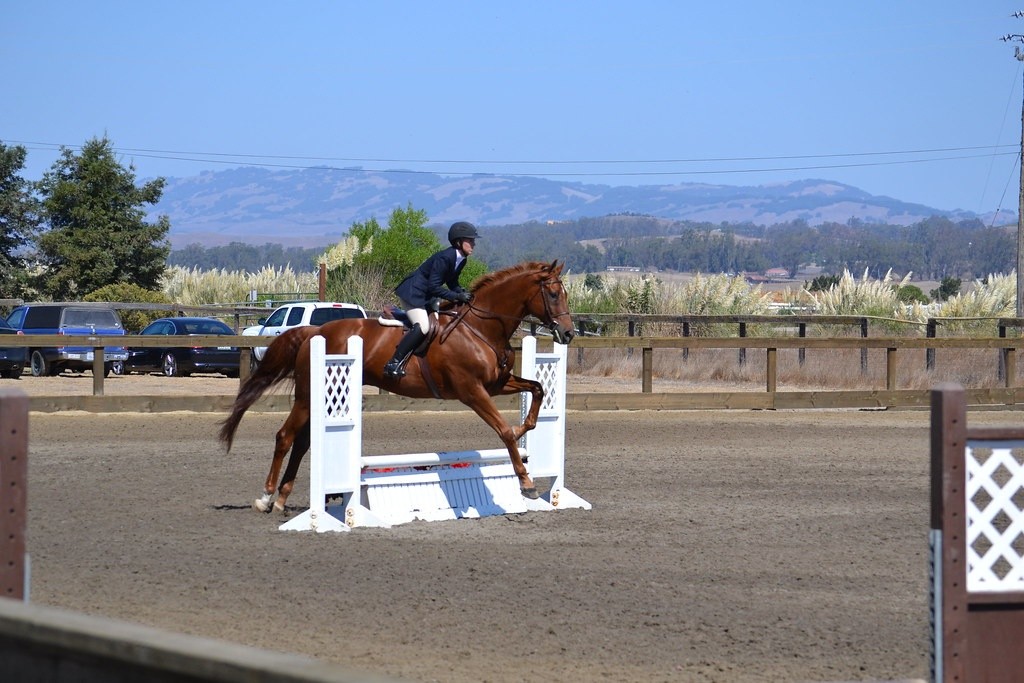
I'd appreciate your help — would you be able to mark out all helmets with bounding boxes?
[447,221,482,239]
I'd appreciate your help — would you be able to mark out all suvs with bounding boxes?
[4,302,129,378]
[240,303,367,374]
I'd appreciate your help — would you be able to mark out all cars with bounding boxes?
[111,317,240,378]
[0,318,27,379]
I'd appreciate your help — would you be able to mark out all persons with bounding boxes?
[380,222,483,377]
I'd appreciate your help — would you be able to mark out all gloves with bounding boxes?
[457,293,473,303]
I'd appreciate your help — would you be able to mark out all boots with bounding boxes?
[383,323,431,376]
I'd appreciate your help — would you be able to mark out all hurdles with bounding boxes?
[276,332,593,533]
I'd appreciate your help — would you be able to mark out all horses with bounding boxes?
[214,258,575,515]
